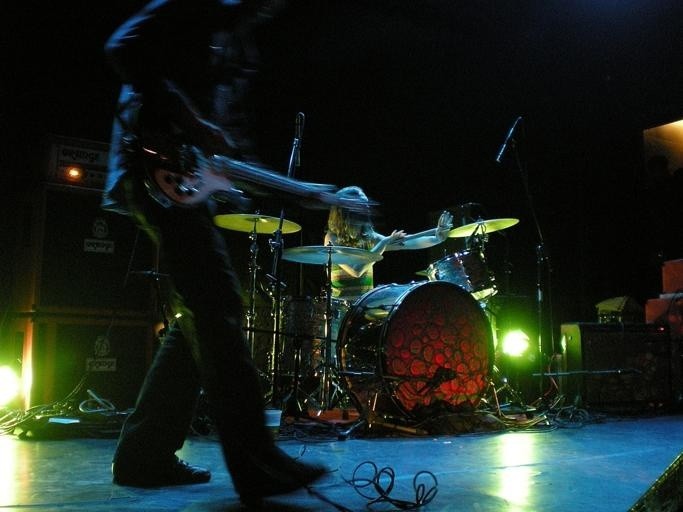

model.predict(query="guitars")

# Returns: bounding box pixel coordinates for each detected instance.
[144,149,380,213]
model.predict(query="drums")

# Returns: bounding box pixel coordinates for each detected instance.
[337,281,495,413]
[426,248,498,302]
[293,298,351,371]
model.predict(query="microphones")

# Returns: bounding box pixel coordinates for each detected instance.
[495,117,521,162]
[294,113,301,166]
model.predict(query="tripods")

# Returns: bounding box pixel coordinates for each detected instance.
[242,229,571,441]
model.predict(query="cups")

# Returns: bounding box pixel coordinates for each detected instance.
[262,408,283,438]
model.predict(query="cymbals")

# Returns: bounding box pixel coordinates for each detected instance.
[446,217,518,238]
[281,245,384,267]
[214,213,301,237]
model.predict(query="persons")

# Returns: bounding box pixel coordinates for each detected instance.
[99,1,328,503]
[322,184,455,363]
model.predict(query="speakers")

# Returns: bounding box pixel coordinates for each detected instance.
[45,317,154,420]
[34,184,155,318]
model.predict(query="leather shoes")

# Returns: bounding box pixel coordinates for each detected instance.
[112,454,211,489]
[236,455,332,504]
